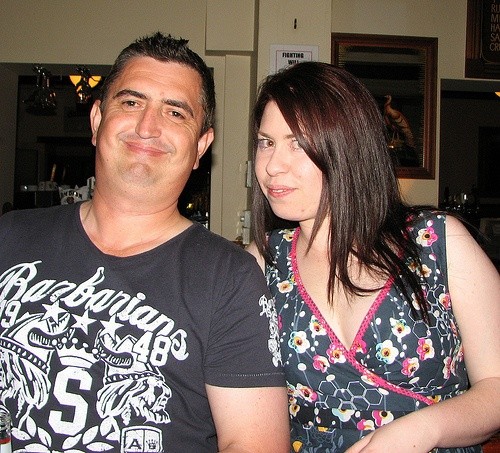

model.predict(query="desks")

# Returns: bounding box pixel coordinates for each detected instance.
[16,190,57,207]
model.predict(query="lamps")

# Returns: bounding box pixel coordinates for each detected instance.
[23,65,58,110]
[69,66,103,114]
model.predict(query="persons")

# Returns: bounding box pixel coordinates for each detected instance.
[247,62,500,453]
[0,31,292,453]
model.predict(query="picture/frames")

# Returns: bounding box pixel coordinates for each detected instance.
[465,0,500,79]
[331,32,436,178]
